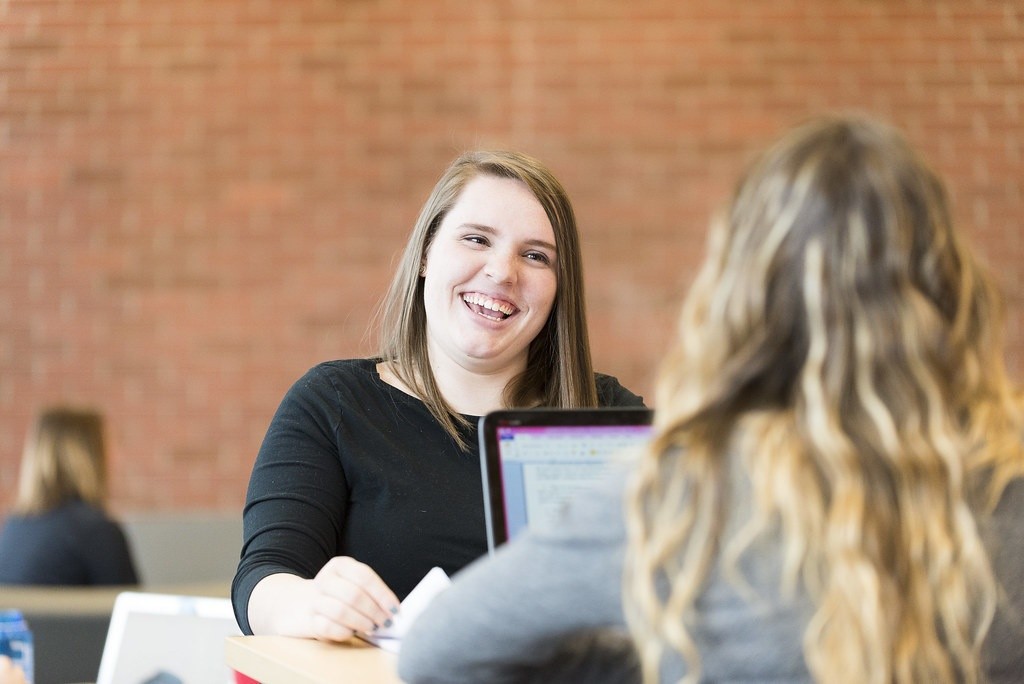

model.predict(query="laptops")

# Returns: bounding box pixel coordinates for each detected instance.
[477,407,655,553]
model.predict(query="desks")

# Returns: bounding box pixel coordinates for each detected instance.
[231,634,404,684]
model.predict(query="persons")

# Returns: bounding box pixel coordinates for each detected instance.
[0,404,144,587]
[394,109,1024,684]
[231,151,653,641]
